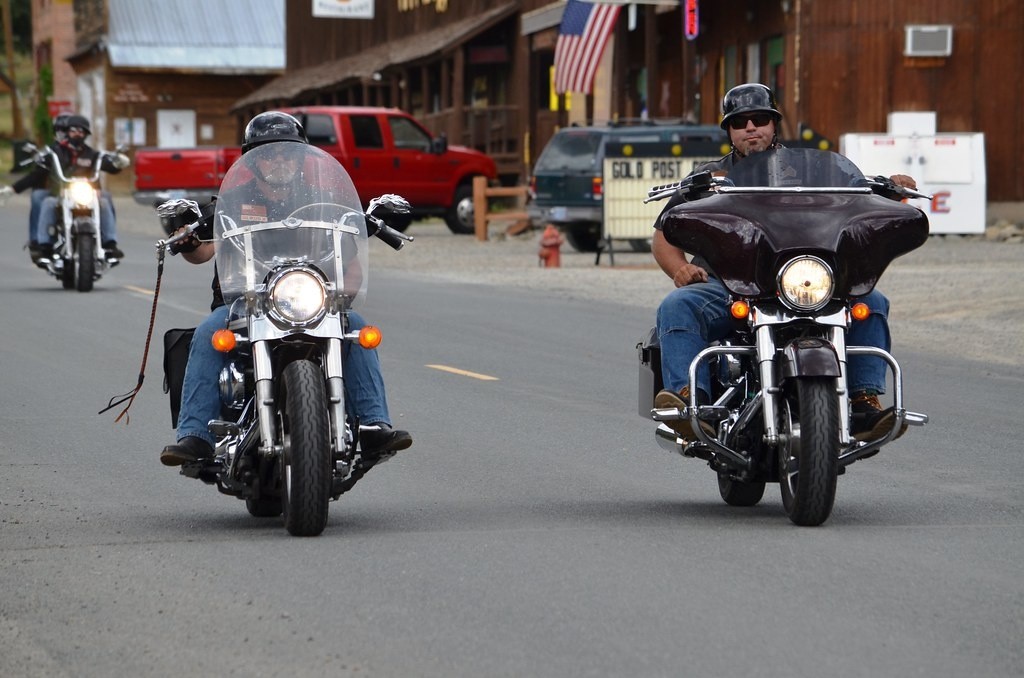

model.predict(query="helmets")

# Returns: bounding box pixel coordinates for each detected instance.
[52,116,70,128]
[241,111,308,155]
[721,83,783,131]
[66,116,90,134]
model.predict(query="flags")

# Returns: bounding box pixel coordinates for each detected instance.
[554,0,622,94]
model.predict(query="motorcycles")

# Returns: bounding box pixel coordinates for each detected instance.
[640,165,931,529]
[151,140,414,537]
[18,140,134,294]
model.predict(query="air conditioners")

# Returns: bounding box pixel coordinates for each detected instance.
[902,24,953,56]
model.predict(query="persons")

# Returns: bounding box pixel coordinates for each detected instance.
[160,111,413,466]
[652,83,916,444]
[0,113,130,263]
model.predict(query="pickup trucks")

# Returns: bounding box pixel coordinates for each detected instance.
[129,103,502,237]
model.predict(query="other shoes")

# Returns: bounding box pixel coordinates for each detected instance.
[30,240,53,262]
[103,241,123,258]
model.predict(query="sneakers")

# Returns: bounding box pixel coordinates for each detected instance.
[850,390,908,442]
[160,436,213,466]
[655,386,716,442]
[359,422,412,455]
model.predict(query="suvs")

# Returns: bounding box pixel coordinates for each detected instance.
[525,115,751,253]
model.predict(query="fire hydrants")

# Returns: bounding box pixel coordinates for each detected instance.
[538,222,565,271]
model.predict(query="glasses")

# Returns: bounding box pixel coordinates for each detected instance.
[54,126,66,132]
[69,126,85,133]
[729,112,772,129]
